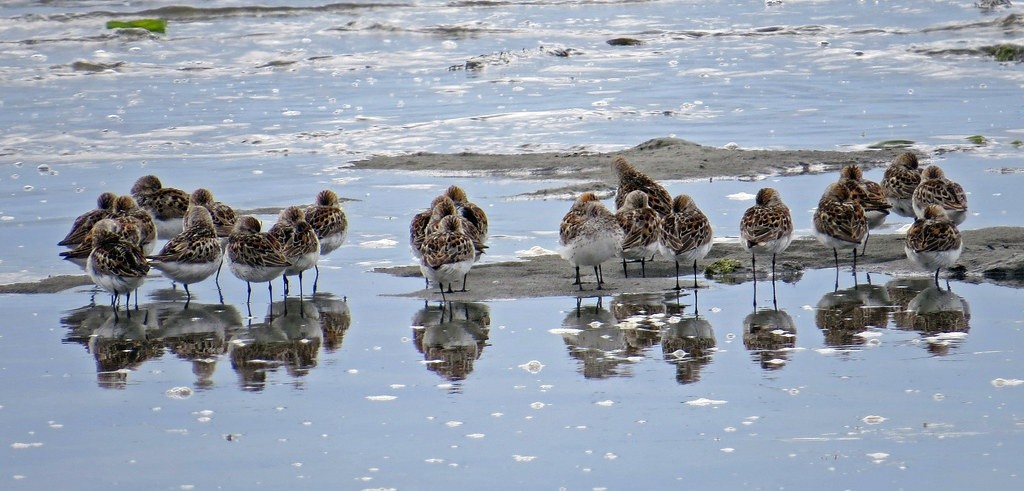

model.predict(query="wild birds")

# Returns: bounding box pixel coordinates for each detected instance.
[61,287,969,389]
[59,154,971,323]
